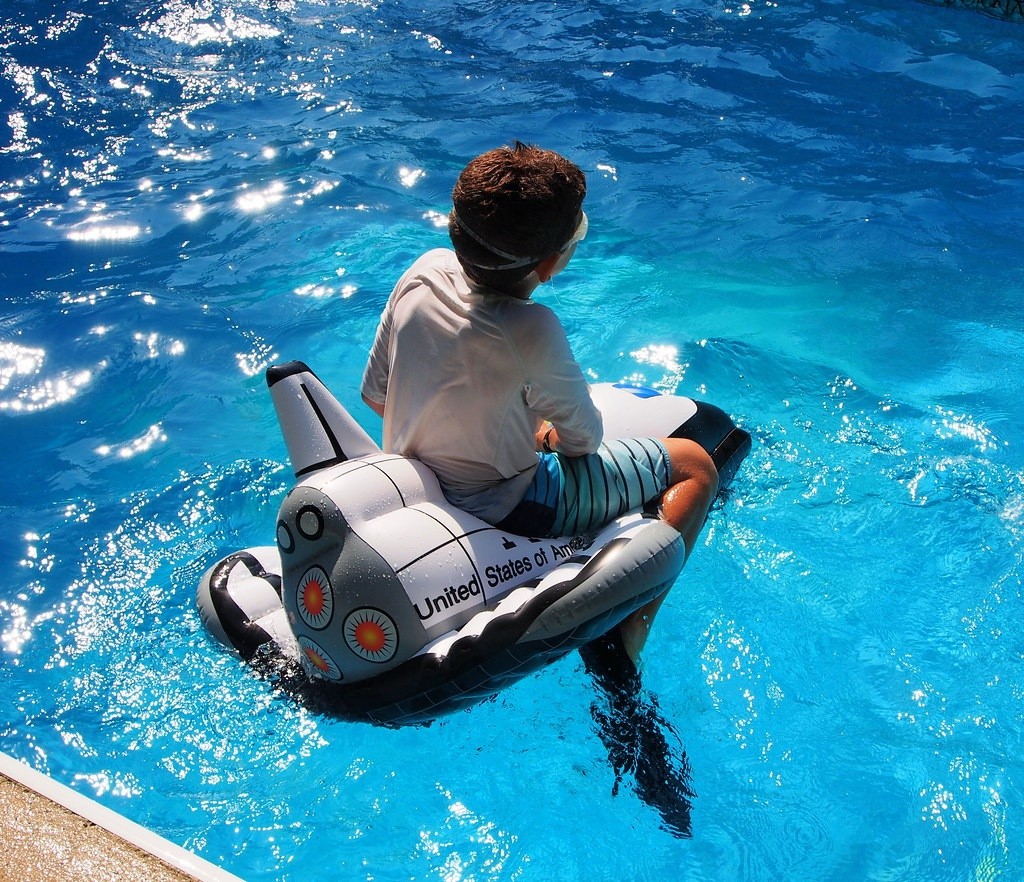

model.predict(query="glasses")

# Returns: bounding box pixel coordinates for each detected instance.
[559,211,588,252]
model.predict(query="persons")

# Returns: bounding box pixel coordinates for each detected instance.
[358,141,721,702]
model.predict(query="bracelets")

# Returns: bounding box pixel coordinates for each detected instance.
[541,424,559,454]
